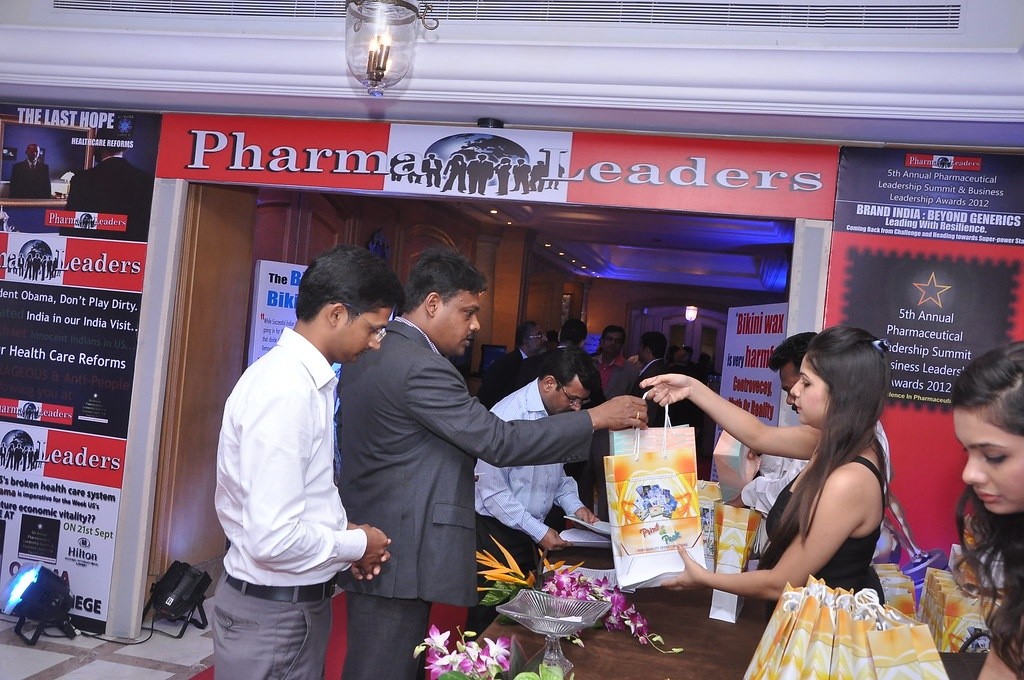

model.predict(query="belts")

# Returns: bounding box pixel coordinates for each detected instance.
[226,574,336,603]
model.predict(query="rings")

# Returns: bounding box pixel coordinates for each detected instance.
[637,412,639,419]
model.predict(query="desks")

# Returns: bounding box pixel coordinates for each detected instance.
[474,549,773,680]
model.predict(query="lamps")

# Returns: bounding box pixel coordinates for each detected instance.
[0,561,77,647]
[343,0,439,97]
[142,560,213,639]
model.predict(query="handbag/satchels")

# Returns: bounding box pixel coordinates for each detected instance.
[708,501,762,624]
[603,391,708,594]
[743,562,1009,680]
[697,479,721,573]
[608,392,696,470]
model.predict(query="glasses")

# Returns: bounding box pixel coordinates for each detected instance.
[330,302,386,342]
[555,375,591,406]
[528,334,542,339]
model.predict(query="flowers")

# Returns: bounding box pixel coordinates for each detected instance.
[412,624,541,680]
[475,533,685,655]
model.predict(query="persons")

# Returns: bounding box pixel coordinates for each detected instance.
[337,246,648,680]
[639,325,893,624]
[58,123,136,237]
[464,346,600,643]
[8,144,51,199]
[951,340,1024,680]
[212,242,406,680]
[476,319,711,533]
